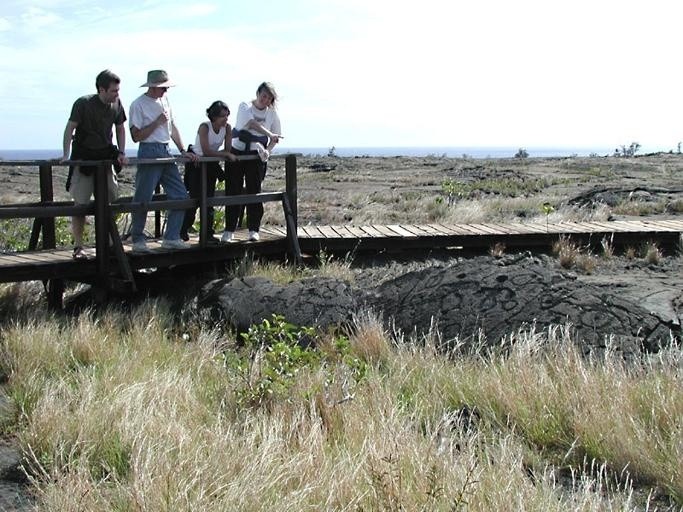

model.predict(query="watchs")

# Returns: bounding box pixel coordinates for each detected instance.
[180,148,186,152]
[120,151,125,155]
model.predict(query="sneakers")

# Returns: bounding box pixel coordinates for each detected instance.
[161,238,192,250]
[220,229,242,244]
[71,246,88,260]
[198,233,219,244]
[131,238,151,254]
[247,230,261,242]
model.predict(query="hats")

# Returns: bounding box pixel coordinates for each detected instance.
[139,70,177,88]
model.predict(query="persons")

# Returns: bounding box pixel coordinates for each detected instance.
[58,70,127,262]
[129,69,200,252]
[220,81,284,244]
[180,100,240,244]
